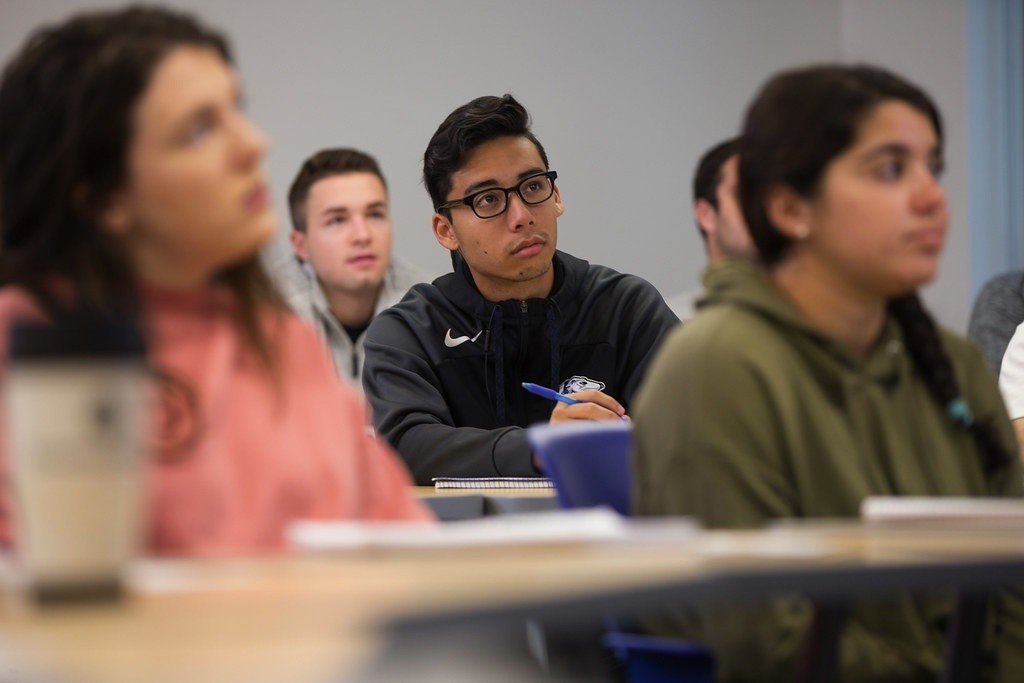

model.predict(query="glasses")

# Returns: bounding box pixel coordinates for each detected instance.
[436,171,557,218]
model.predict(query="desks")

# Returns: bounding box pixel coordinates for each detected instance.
[0,488,1024,683]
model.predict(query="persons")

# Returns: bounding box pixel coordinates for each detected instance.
[636,64,1024,683]
[0,7,439,565]
[277,90,1024,487]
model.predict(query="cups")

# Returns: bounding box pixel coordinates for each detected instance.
[7,358,147,606]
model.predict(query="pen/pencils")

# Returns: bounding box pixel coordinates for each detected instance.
[521,381,578,405]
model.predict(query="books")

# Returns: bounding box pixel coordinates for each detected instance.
[430,476,554,489]
